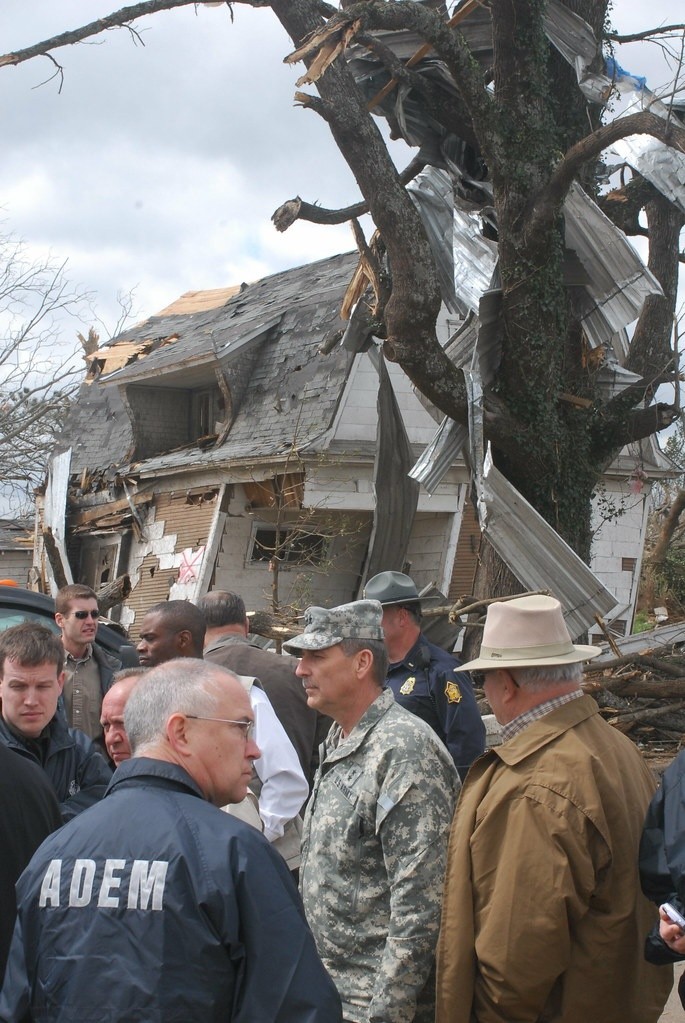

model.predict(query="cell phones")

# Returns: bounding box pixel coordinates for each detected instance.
[662,903,685,938]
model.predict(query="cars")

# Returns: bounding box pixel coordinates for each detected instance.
[0,580,140,656]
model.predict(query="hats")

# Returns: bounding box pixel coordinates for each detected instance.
[283,598,384,656]
[453,595,602,672]
[363,571,440,606]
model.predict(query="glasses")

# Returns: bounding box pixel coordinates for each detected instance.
[474,669,520,689]
[168,715,254,742]
[61,610,100,619]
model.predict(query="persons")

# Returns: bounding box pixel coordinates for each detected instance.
[434,596,675,1023]
[0,622,113,825]
[282,598,463,1023]
[0,657,343,1023]
[100,667,153,768]
[195,591,316,782]
[135,600,310,876]
[54,583,127,752]
[362,570,488,772]
[636,747,685,1008]
